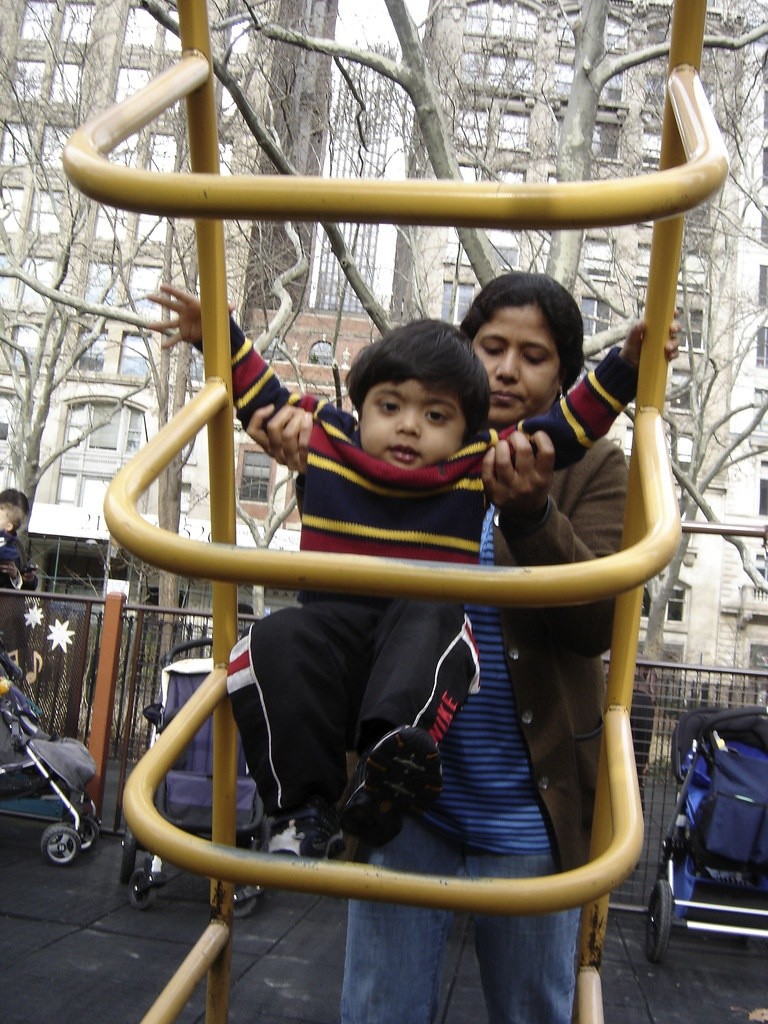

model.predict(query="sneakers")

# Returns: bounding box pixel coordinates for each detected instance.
[260,804,347,862]
[339,725,444,851]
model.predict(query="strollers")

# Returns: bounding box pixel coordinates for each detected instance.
[126,638,276,919]
[643,706,768,966]
[0,626,103,866]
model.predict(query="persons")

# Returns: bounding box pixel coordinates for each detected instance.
[146,283,679,864]
[0,488,38,693]
[247,273,629,1024]
